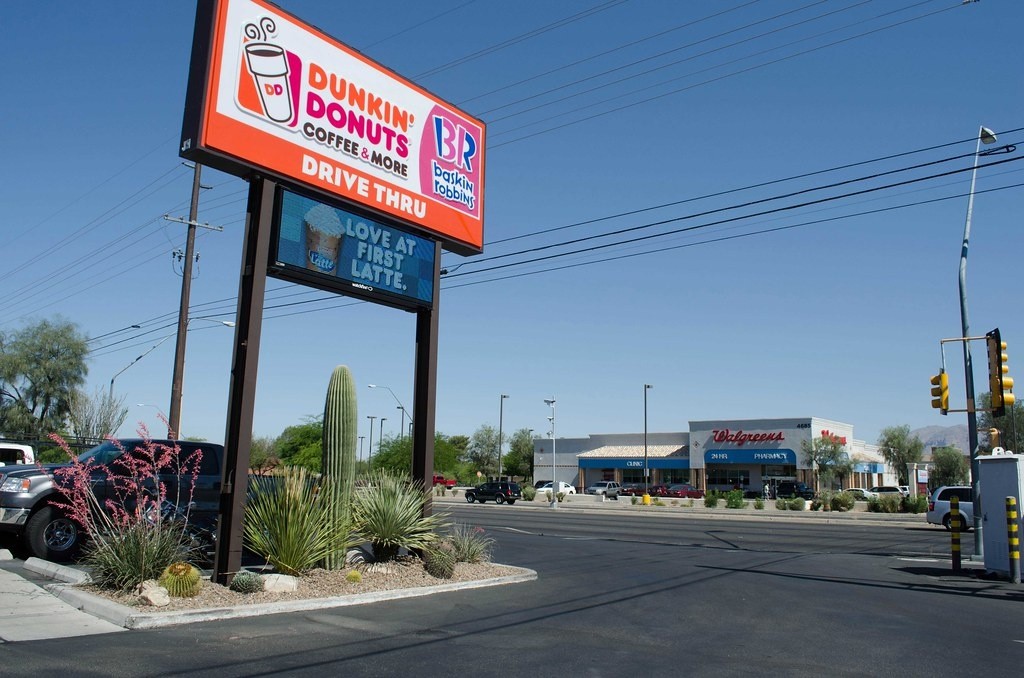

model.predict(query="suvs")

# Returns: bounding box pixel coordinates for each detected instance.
[465,480,521,505]
[776,481,814,500]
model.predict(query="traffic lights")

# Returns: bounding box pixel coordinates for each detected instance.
[985,328,1015,418]
[930,374,949,410]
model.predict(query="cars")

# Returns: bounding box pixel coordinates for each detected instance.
[843,485,909,501]
[622,484,705,498]
[926,486,974,531]
[584,481,622,500]
[535,480,576,497]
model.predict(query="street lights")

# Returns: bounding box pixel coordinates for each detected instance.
[644,383,653,497]
[498,394,509,482]
[397,407,403,441]
[957,125,998,556]
[379,418,387,451]
[358,436,365,467]
[367,384,413,421]
[109,316,236,411]
[367,415,377,466]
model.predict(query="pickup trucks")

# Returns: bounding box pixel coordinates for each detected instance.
[433,475,457,489]
[0,439,320,566]
